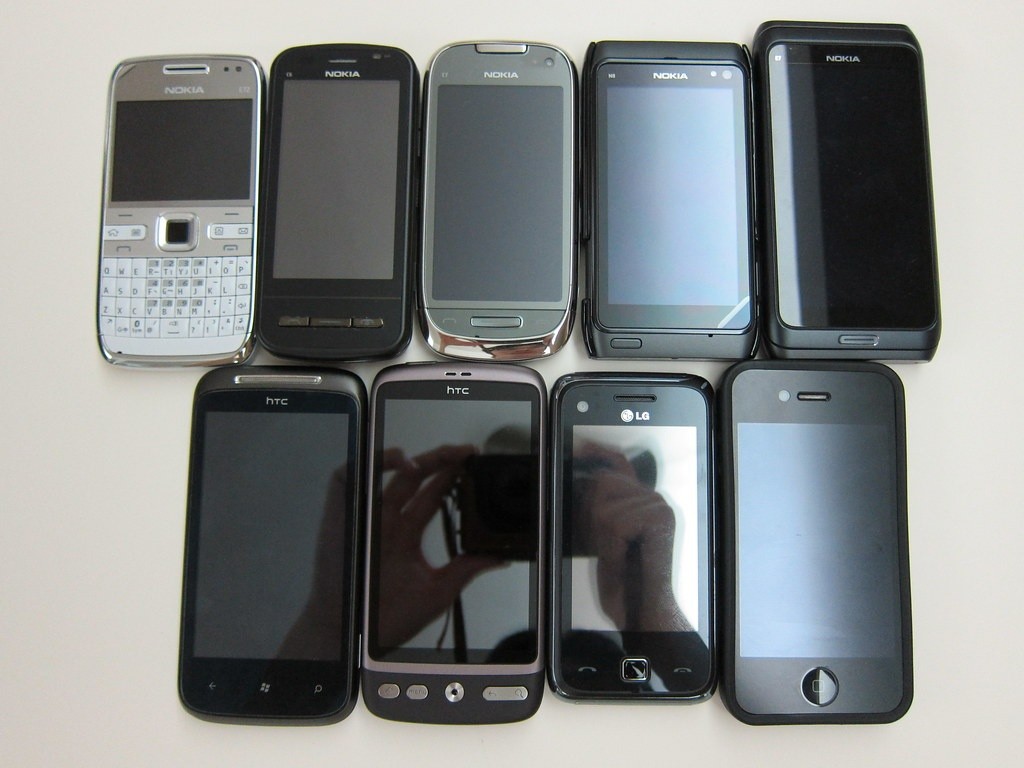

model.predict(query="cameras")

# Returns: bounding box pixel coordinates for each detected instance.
[454,452,602,563]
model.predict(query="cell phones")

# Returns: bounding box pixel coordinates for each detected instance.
[95,20,941,726]
